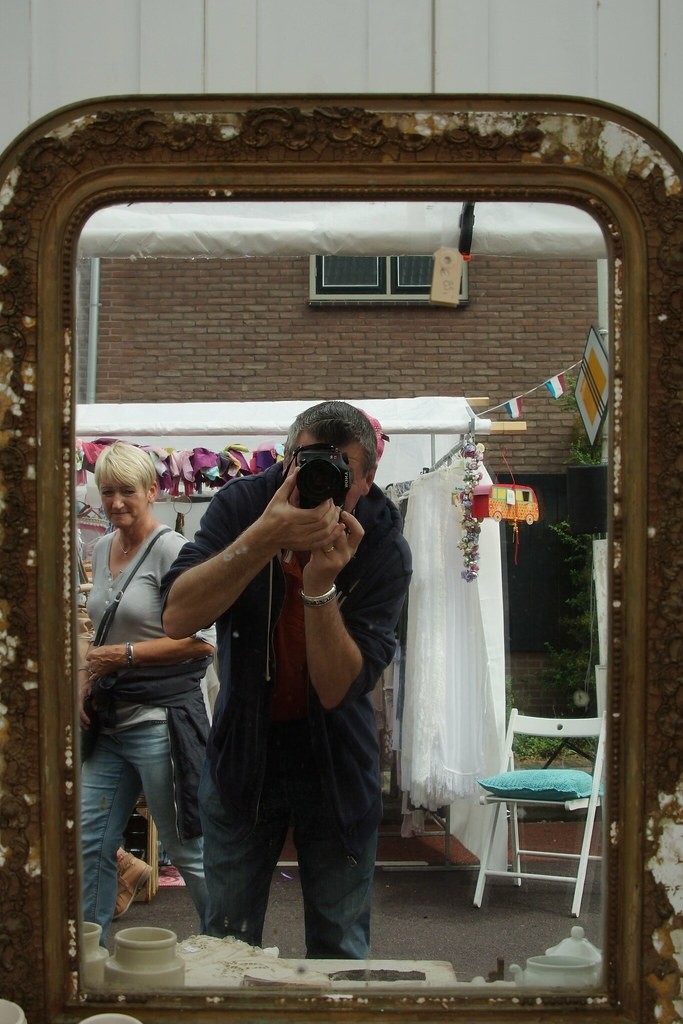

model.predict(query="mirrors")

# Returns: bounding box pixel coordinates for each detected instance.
[0,92,683,1024]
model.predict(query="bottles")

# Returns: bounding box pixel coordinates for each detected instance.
[510,956,599,987]
[78,1012,142,1024]
[0,999,28,1024]
[82,922,109,989]
[103,926,185,990]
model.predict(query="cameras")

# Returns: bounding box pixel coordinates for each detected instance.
[296,444,353,523]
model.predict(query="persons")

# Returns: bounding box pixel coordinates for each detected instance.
[74,441,218,956]
[159,401,414,960]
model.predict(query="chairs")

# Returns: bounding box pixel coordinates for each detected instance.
[472,708,606,919]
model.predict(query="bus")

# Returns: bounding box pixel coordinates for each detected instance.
[471,483,540,526]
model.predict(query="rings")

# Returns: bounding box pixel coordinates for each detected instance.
[328,546,334,552]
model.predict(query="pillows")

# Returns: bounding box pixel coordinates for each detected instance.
[476,768,604,800]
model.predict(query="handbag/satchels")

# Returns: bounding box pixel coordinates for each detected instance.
[80,687,114,747]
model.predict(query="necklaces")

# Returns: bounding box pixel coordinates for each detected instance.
[118,539,137,555]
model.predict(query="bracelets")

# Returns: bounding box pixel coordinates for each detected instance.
[301,584,337,607]
[127,642,134,668]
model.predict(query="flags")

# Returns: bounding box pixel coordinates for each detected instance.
[545,374,566,400]
[504,396,522,419]
